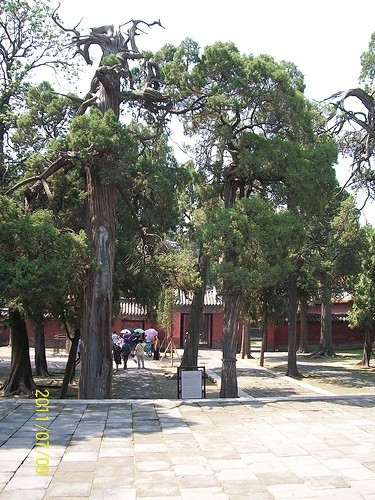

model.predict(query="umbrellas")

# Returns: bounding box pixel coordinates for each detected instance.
[134,329,144,334]
[145,328,159,337]
[120,329,131,334]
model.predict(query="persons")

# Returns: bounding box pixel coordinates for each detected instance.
[77,332,162,371]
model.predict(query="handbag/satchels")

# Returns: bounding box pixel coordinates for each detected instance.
[133,356,137,363]
